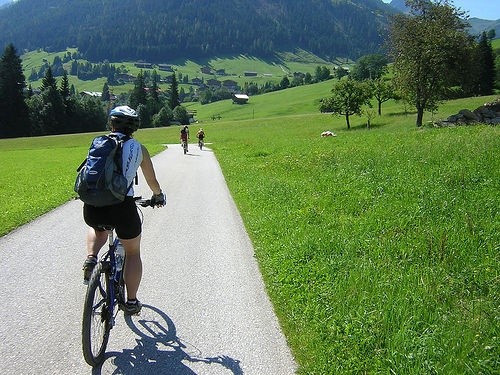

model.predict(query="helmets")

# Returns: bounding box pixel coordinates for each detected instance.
[109,105,140,130]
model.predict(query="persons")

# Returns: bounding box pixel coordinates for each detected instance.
[196,128,206,147]
[81,106,166,316]
[180,125,190,152]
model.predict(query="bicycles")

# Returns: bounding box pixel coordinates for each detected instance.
[181,138,190,154]
[72,194,167,366]
[198,137,204,151]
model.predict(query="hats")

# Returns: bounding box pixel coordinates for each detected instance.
[185,126,188,128]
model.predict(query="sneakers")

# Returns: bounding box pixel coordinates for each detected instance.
[125,300,142,315]
[83,255,97,281]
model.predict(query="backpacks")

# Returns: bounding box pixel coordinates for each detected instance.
[74,133,133,206]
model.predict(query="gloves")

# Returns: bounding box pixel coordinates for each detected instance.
[150,190,167,209]
